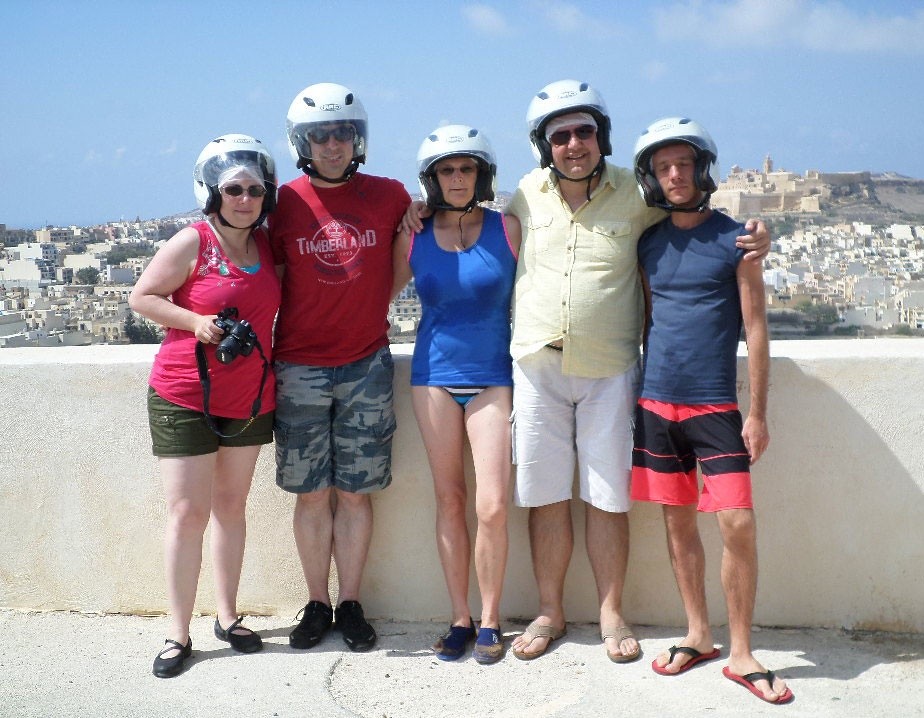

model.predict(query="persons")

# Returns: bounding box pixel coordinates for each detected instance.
[396,80,771,662]
[128,134,284,680]
[267,84,411,652]
[632,118,793,705]
[389,124,521,662]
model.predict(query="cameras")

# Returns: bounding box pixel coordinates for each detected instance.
[214,315,258,365]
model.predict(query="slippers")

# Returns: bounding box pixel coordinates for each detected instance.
[722,663,793,704]
[598,625,640,663]
[511,620,568,661]
[651,642,722,676]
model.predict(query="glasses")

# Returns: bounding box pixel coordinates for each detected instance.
[547,125,596,145]
[222,184,267,197]
[436,164,480,176]
[305,126,354,144]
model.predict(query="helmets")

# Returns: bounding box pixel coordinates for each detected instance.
[285,83,368,163]
[525,79,613,169]
[632,118,718,207]
[416,126,495,208]
[192,134,279,216]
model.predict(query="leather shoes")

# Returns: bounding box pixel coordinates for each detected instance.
[213,613,264,654]
[153,638,193,679]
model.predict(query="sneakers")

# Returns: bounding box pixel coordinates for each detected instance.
[473,624,505,665]
[289,601,334,649]
[334,600,377,652]
[436,615,477,660]
